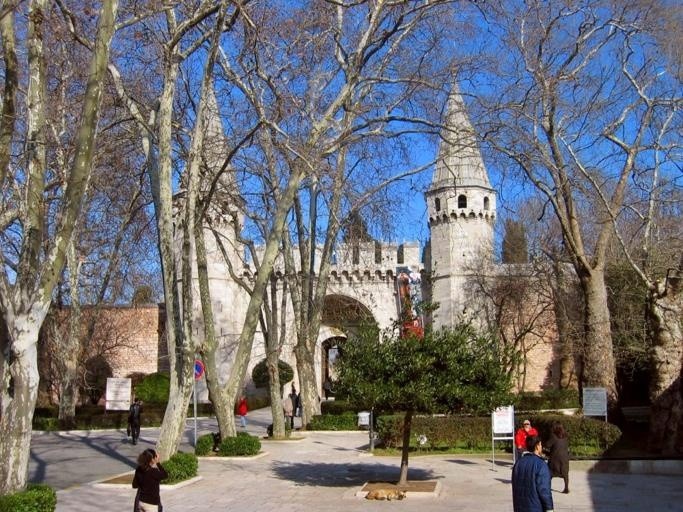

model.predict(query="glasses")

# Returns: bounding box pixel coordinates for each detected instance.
[523,424,529,425]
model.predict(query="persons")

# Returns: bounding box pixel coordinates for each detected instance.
[236,390,248,429]
[126,397,143,446]
[291,381,296,394]
[296,392,302,417]
[513,419,538,461]
[509,435,555,512]
[543,420,571,496]
[283,393,296,429]
[128,448,167,512]
[280,395,285,408]
[289,389,297,418]
[321,378,330,401]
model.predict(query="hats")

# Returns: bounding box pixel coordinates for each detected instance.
[133,398,140,402]
[523,420,530,424]
